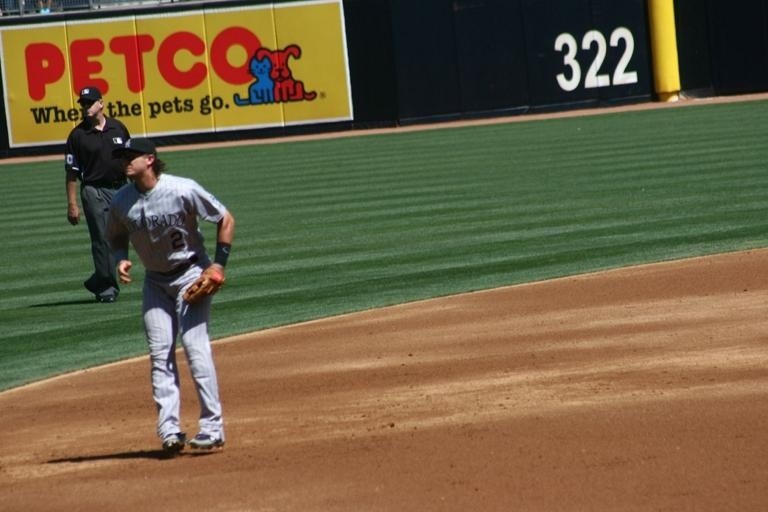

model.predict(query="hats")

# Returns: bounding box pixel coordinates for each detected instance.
[78,86,100,104]
[111,137,157,160]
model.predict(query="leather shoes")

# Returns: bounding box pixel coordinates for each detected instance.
[97,295,116,303]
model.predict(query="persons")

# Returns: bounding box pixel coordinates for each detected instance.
[63,86,131,304]
[105,136,237,458]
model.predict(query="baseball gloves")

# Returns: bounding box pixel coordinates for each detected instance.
[183,264,225,304]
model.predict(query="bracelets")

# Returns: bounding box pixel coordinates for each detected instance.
[213,240,232,267]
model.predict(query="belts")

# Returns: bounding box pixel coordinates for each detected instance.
[176,255,197,270]
[94,179,128,189]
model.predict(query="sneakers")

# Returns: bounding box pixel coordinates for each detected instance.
[163,438,185,452]
[190,435,224,450]
